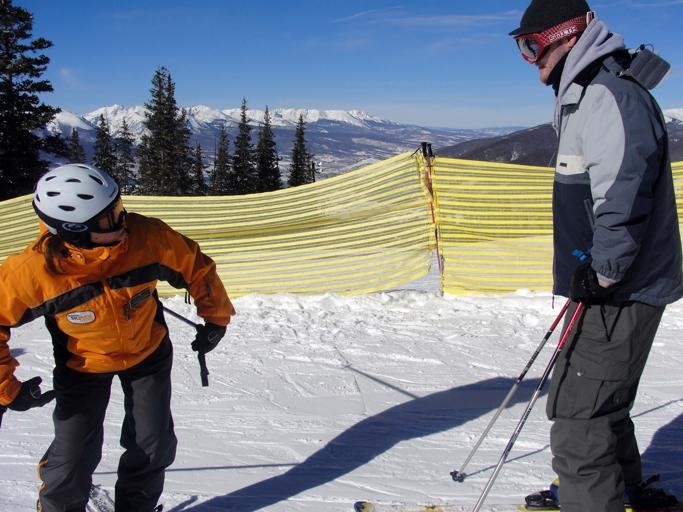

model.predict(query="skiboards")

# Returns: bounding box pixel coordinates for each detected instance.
[354,473,683,512]
[85,482,116,512]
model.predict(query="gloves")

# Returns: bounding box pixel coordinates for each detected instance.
[6,376,56,411]
[568,256,609,307]
[191,323,226,356]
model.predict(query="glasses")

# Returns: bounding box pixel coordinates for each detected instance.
[33,188,127,234]
[513,11,596,64]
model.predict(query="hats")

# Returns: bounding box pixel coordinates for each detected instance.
[509,0,590,36]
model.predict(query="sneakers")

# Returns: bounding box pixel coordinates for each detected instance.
[526,478,561,507]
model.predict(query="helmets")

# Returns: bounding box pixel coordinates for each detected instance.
[31,162,119,249]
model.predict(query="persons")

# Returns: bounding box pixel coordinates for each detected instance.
[509,1,682,510]
[0,164,237,508]
